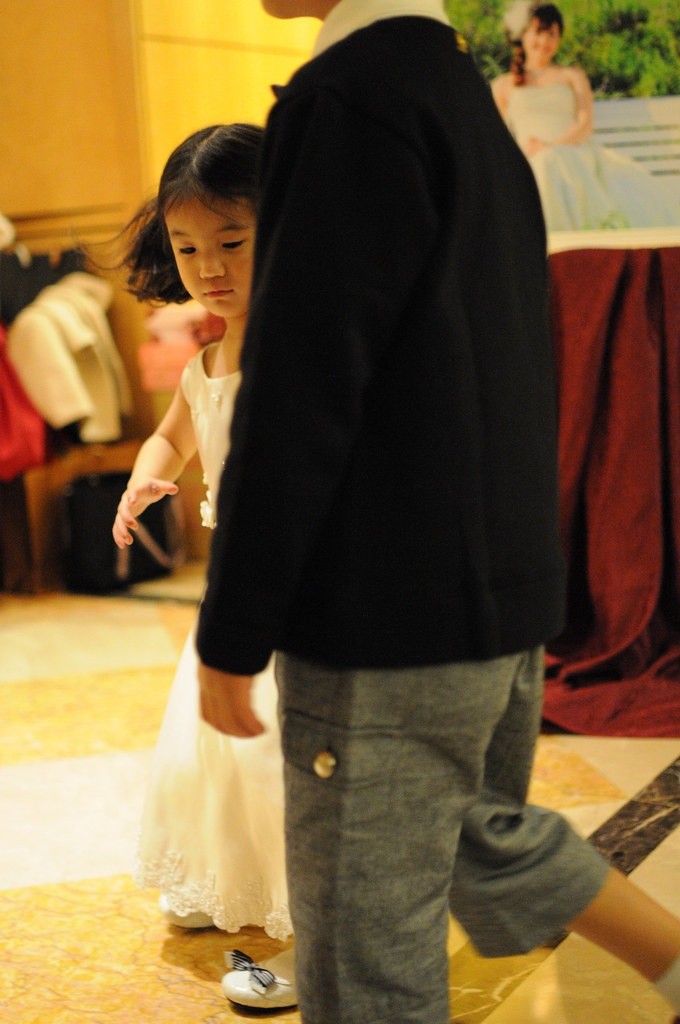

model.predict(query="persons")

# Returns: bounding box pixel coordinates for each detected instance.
[195,0,680,1024]
[111,123,299,1007]
[490,0,680,230]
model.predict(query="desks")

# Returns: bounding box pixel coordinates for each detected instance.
[546,232,680,742]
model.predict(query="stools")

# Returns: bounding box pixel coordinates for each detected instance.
[12,436,186,593]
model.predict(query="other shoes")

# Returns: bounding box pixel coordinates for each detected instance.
[221,949,297,1008]
[160,895,215,928]
